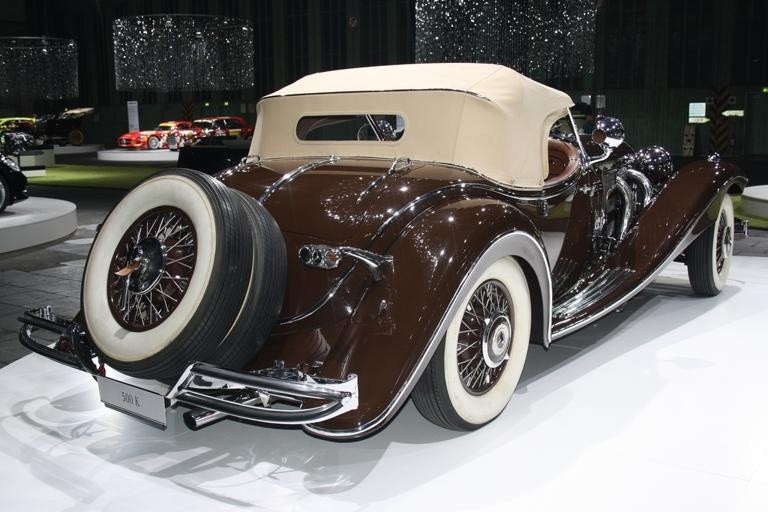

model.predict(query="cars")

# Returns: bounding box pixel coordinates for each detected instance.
[8,60,754,449]
[0,151,30,213]
[0,106,252,154]
